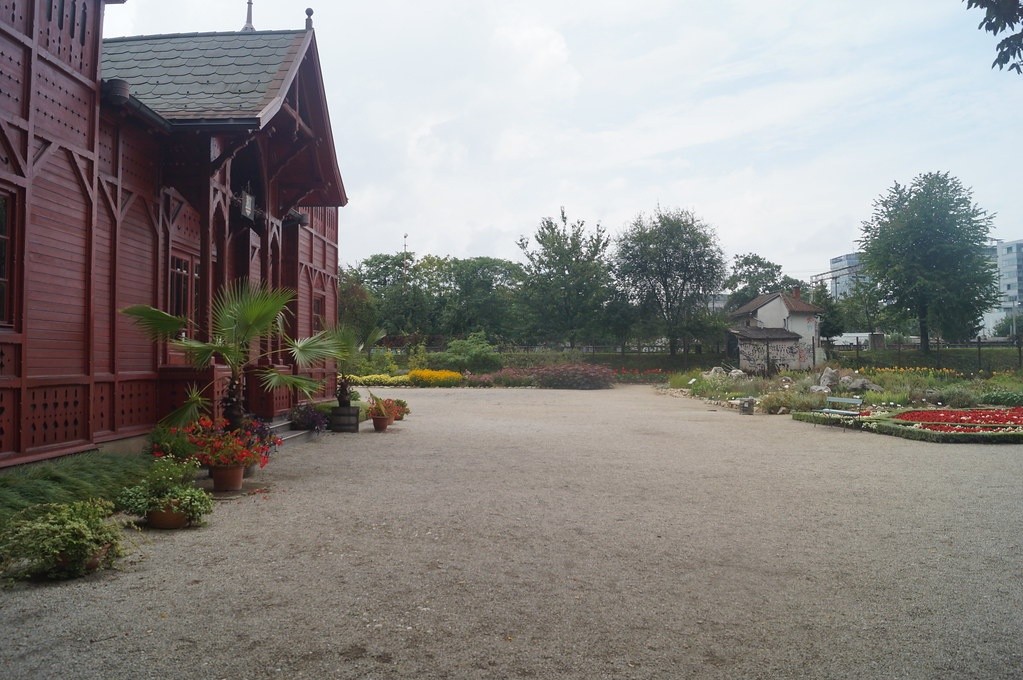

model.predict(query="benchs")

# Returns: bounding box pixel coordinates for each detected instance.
[811,397,864,433]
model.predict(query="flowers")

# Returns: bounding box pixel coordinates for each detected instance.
[365,399,411,418]
[115,453,214,527]
[153,417,284,469]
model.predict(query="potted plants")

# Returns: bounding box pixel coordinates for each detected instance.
[316,316,393,425]
[0,497,155,591]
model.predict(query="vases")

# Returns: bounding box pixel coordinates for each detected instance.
[387,416,394,424]
[394,413,403,420]
[147,499,190,529]
[371,416,389,431]
[210,464,246,491]
